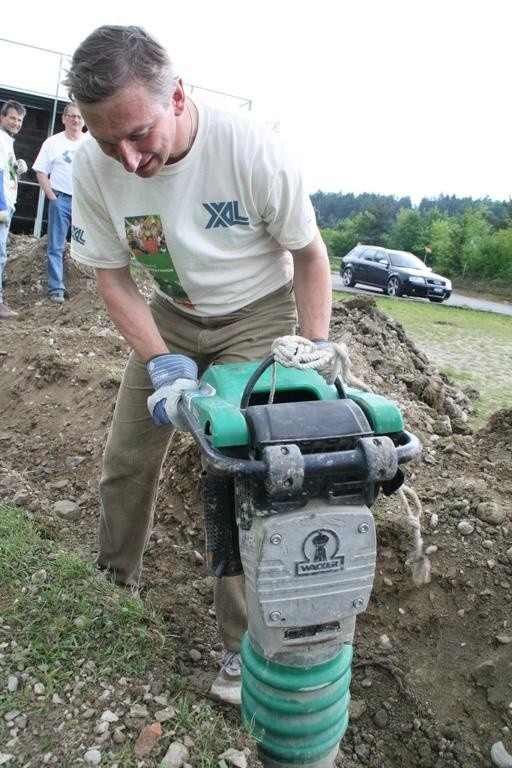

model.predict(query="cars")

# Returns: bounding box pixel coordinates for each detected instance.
[340,245,453,303]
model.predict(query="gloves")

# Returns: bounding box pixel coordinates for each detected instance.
[143,352,199,433]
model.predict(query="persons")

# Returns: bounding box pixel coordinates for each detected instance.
[32,102,95,308]
[59,22,335,708]
[0,97,29,320]
[122,214,170,257]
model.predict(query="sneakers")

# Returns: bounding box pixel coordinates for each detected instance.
[48,289,65,307]
[0,303,21,322]
[207,648,245,709]
